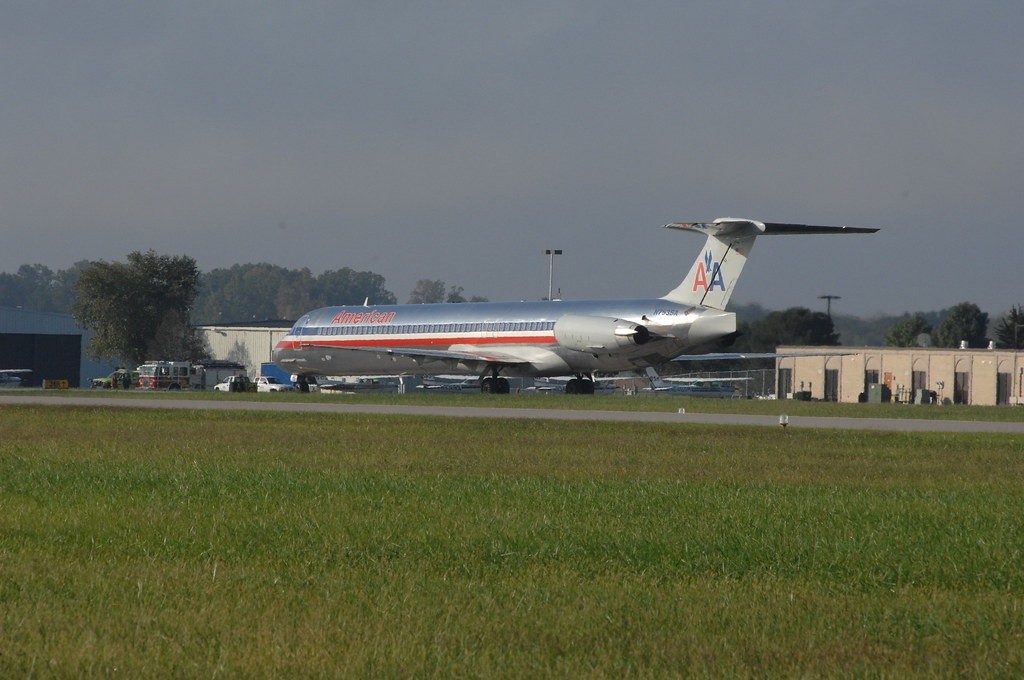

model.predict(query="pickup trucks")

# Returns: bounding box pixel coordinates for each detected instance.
[214,375,257,394]
[253,375,295,393]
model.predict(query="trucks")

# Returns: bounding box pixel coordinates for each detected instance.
[136,360,207,392]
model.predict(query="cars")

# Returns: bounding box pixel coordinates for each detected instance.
[90,368,141,390]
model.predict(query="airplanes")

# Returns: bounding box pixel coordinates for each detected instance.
[270,215,883,396]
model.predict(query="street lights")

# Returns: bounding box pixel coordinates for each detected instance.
[546,250,562,300]
